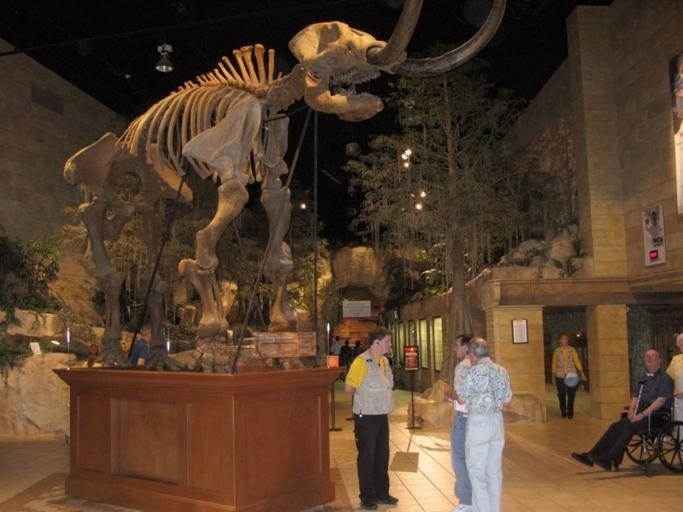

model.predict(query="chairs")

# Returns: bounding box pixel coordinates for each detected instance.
[378,495,398,504]
[360,500,378,510]
[587,453,612,471]
[572,451,594,467]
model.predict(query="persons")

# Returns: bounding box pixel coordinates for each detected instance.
[128,323,151,368]
[343,326,398,509]
[338,339,351,381]
[444,333,472,512]
[570,348,673,474]
[643,210,662,237]
[329,335,342,355]
[461,336,514,511]
[353,339,363,359]
[665,333,683,470]
[550,332,589,419]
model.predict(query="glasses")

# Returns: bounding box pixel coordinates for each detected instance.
[151,34,174,73]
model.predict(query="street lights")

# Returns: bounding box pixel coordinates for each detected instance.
[563,373,580,387]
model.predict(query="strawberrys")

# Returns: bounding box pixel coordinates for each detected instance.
[620,392,683,474]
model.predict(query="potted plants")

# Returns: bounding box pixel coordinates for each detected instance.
[666,47,683,214]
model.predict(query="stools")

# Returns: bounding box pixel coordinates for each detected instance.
[462,413,467,417]
